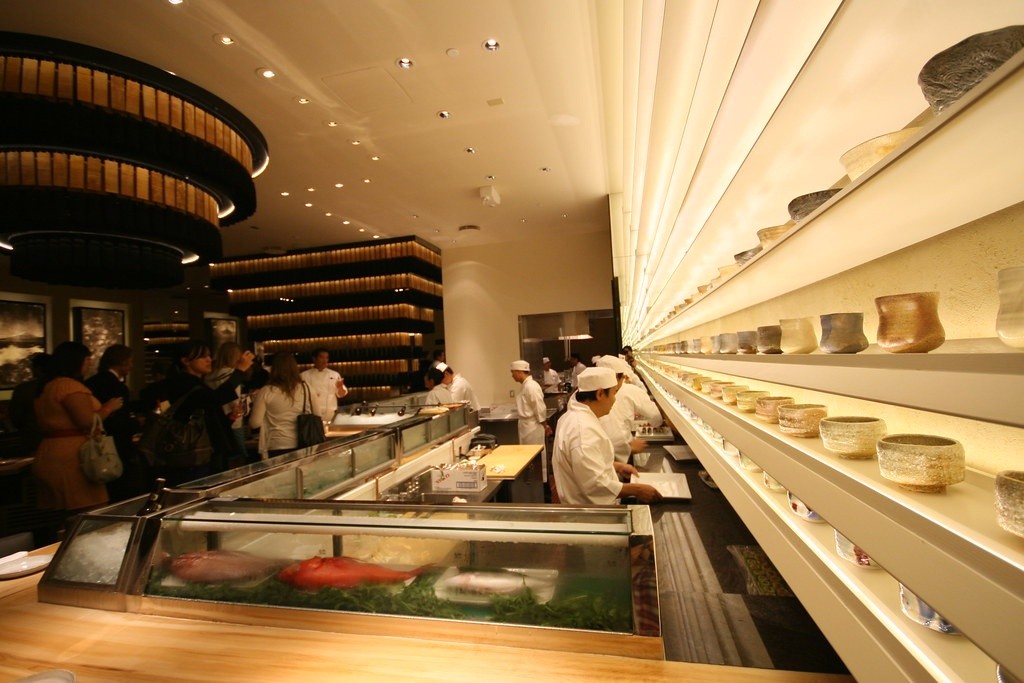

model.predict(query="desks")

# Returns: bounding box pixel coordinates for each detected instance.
[475,439,545,504]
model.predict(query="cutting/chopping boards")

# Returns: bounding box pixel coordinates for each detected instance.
[475,444,544,477]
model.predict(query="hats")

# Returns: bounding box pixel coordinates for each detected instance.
[577,365,618,393]
[598,355,627,373]
[592,356,601,364]
[511,360,530,372]
[543,357,550,364]
[436,362,448,373]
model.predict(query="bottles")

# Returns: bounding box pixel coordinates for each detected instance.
[351,401,407,417]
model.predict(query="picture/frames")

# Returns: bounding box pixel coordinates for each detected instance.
[205,319,238,361]
[72,306,126,379]
[0,300,47,387]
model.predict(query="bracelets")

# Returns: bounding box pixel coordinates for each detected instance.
[102,406,112,413]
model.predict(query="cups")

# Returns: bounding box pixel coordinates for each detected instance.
[819,313,869,354]
[687,326,782,354]
[995,265,1024,348]
[779,317,818,355]
[875,292,945,353]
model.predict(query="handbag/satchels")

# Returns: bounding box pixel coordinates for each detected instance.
[156,388,212,466]
[79,414,124,485]
[297,380,326,448]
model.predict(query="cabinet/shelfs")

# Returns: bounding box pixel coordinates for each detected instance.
[620,0,1023,683]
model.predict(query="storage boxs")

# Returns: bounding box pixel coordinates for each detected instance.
[432,460,489,491]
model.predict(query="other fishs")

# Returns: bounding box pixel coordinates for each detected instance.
[275,554,444,595]
[443,569,556,597]
[169,548,305,582]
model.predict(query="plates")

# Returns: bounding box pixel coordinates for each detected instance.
[631,416,675,441]
[629,472,692,499]
[16,669,77,683]
[0,556,53,578]
[663,445,697,460]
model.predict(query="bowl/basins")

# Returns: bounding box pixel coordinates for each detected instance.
[876,432,966,493]
[778,403,827,438]
[635,453,650,467]
[673,370,793,424]
[734,247,769,268]
[995,470,1024,539]
[788,188,849,223]
[840,126,931,182]
[756,225,800,250]
[674,264,740,313]
[818,414,888,458]
[917,25,1024,118]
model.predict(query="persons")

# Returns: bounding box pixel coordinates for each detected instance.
[510,360,552,504]
[436,362,481,411]
[570,350,587,386]
[542,357,563,392]
[428,350,445,368]
[8,342,349,524]
[424,368,451,404]
[551,346,665,505]
